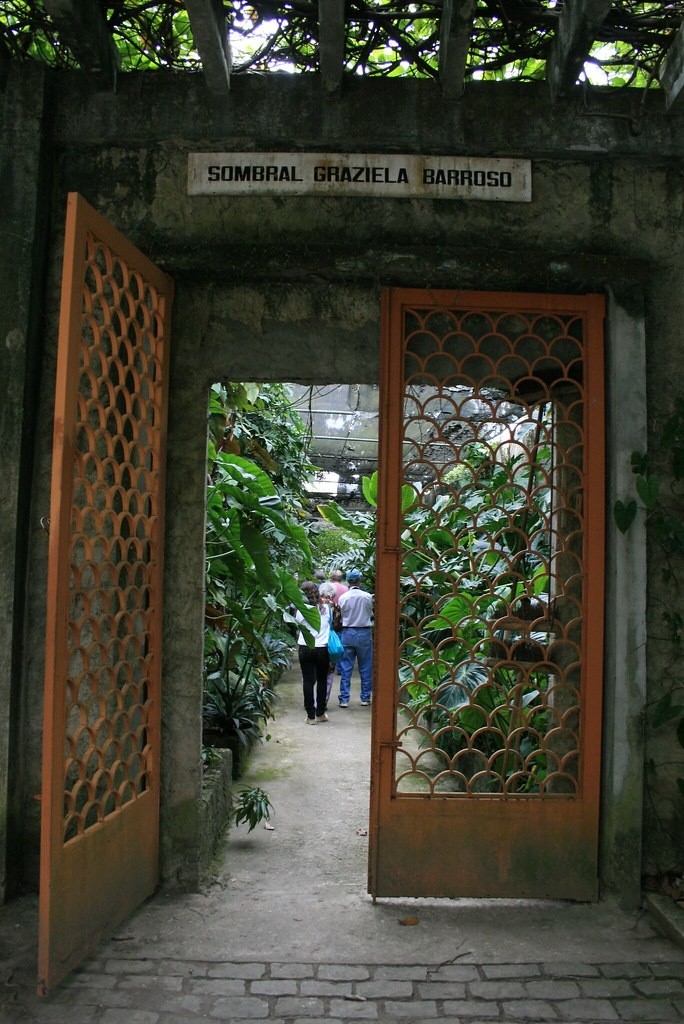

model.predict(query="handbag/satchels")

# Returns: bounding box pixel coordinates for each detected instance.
[326,604,344,662]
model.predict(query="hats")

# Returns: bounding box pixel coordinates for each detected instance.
[346,568,362,583]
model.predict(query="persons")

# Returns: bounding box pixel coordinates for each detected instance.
[290,568,348,723]
[338,569,374,708]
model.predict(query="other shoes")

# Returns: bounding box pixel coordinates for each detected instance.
[316,714,329,721]
[305,718,316,725]
[339,703,348,707]
[361,702,371,706]
